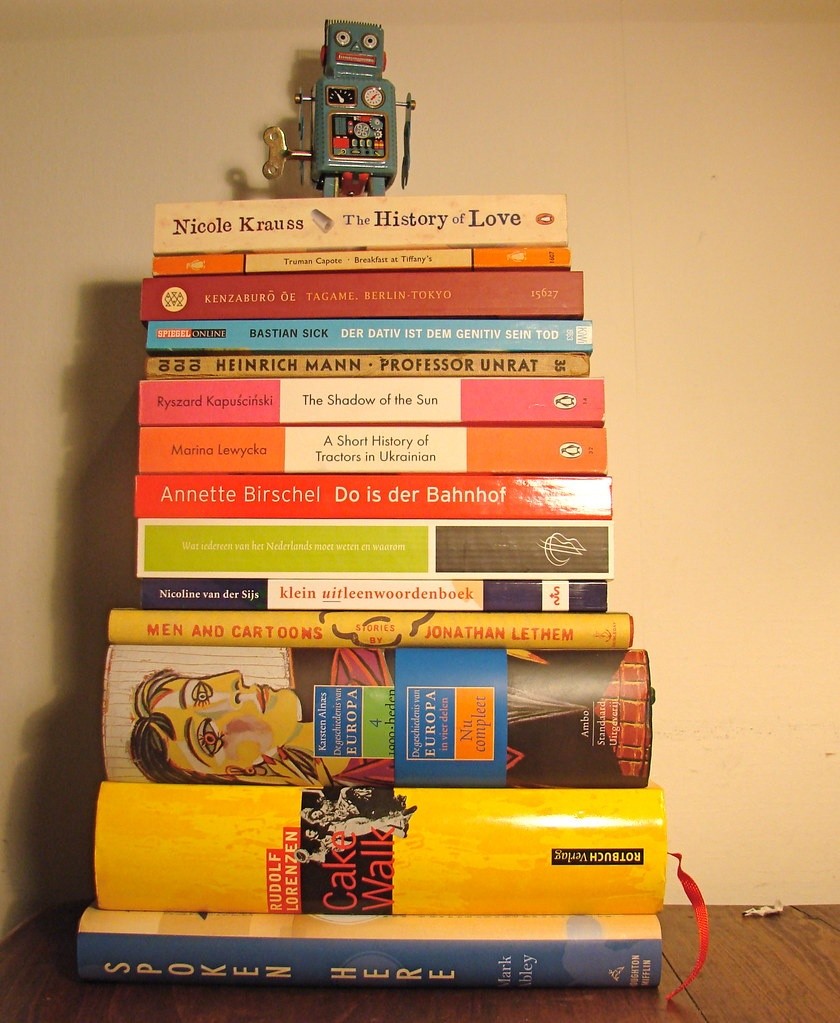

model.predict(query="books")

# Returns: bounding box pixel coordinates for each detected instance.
[76,193,667,987]
